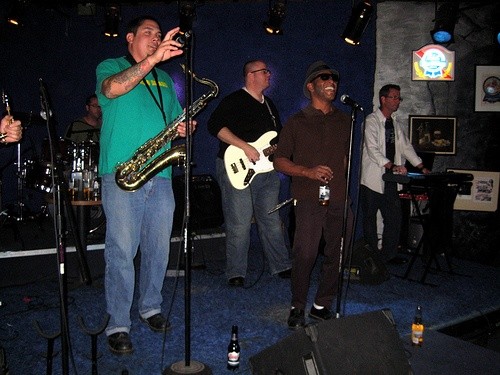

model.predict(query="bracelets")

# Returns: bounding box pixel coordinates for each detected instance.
[420,165,427,169]
[390,164,397,171]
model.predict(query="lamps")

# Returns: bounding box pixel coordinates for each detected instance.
[176,0,197,36]
[344,0,375,46]
[262,0,288,35]
[430,0,461,44]
[103,4,123,37]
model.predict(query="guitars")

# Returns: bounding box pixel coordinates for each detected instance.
[223,130,278,191]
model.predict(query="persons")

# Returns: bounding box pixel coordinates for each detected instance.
[62,97,101,153]
[273,60,353,330]
[207,60,292,286]
[0,114,23,143]
[95,16,197,355]
[358,84,430,265]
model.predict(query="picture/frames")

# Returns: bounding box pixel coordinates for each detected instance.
[473,65,500,112]
[406,114,456,155]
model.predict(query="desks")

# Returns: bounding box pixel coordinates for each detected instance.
[71,198,100,282]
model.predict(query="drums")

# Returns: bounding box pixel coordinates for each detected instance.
[66,171,102,206]
[24,155,60,194]
[60,139,96,173]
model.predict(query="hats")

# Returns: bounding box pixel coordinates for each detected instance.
[303,61,339,99]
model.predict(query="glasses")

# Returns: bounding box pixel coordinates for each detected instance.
[251,69,272,75]
[88,104,101,108]
[385,95,404,102]
[309,72,339,83]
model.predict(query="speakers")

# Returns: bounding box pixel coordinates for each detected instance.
[248,307,415,375]
[173,174,225,232]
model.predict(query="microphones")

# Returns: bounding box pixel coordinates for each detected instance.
[66,122,74,138]
[176,29,194,47]
[341,94,363,110]
[39,78,52,120]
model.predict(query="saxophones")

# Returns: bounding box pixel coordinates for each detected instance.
[114,62,219,191]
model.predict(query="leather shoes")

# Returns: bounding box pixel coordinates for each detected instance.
[146,314,171,331]
[382,246,412,255]
[278,268,292,279]
[108,333,132,353]
[309,304,335,320]
[386,256,409,265]
[287,307,305,329]
[229,277,244,287]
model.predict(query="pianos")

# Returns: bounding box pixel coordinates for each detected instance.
[382,171,475,289]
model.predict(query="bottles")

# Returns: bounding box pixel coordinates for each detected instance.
[412,306,425,348]
[227,325,240,371]
[318,177,330,207]
